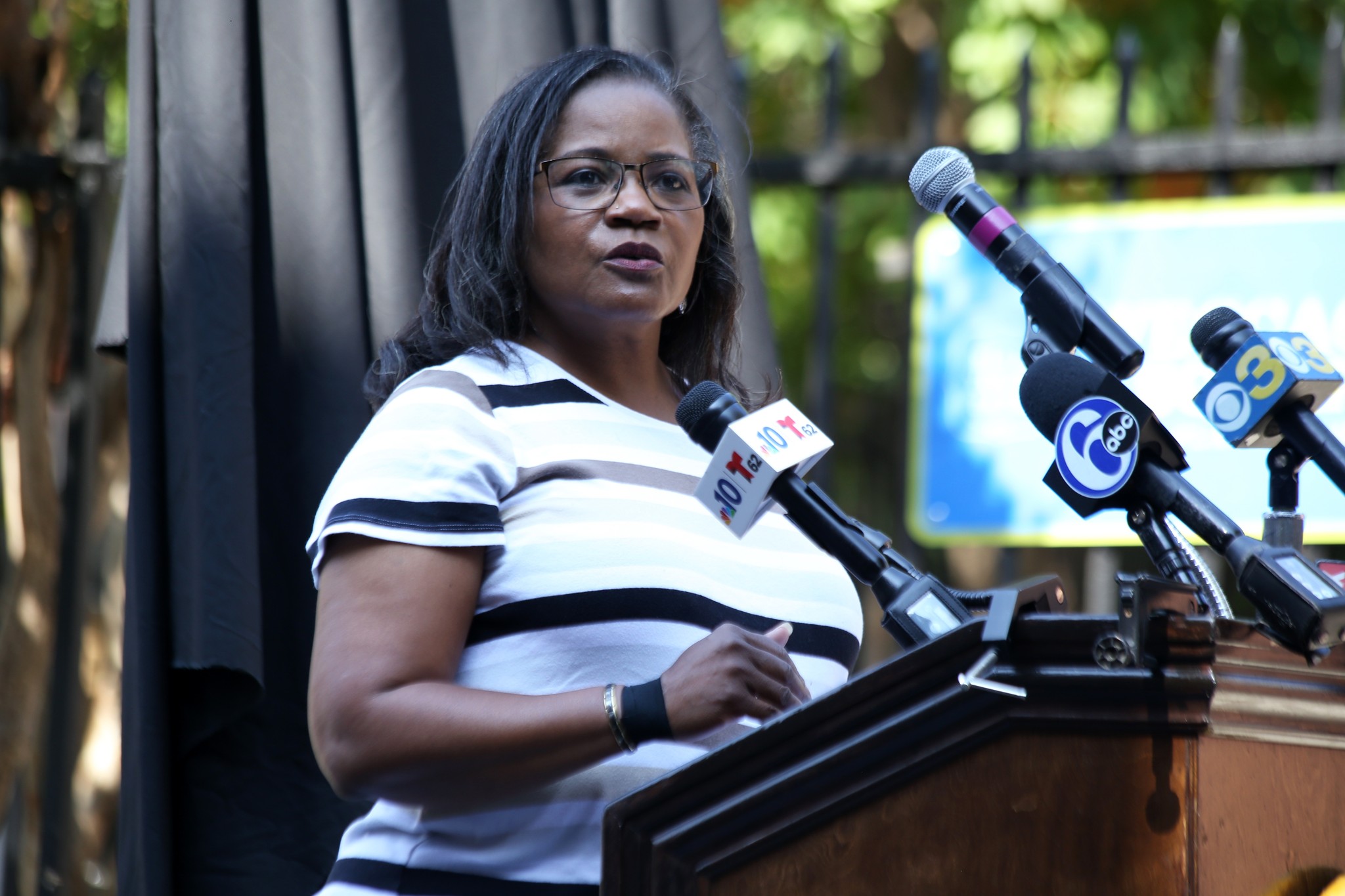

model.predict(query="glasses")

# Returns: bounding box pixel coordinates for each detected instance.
[534,157,718,211]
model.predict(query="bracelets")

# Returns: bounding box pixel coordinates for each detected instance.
[603,683,638,756]
[620,677,676,748]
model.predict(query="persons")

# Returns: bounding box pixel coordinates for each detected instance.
[304,45,866,896]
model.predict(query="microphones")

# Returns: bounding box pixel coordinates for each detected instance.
[908,144,1144,380]
[1019,352,1345,658]
[674,380,974,653]
[1191,306,1345,494]
